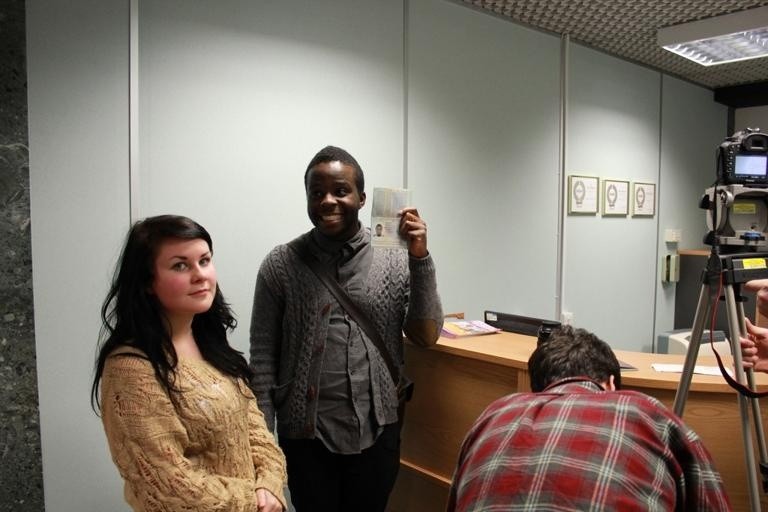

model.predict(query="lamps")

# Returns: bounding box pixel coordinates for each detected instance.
[656,5,767,66]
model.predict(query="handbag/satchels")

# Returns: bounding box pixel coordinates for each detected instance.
[393,364,414,427]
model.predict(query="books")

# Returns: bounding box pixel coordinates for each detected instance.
[441,321,501,338]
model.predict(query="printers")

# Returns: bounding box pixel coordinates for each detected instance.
[658,329,732,358]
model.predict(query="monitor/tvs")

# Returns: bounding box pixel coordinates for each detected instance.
[484,310,560,337]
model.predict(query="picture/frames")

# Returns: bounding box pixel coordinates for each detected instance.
[601,179,629,216]
[567,174,598,214]
[630,181,655,216]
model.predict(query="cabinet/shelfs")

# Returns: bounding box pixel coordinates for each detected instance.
[674,250,767,337]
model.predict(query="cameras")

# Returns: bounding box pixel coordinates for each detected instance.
[714,126,768,189]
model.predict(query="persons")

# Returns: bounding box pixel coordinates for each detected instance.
[739,278,768,373]
[250,146,444,511]
[101,215,288,511]
[446,325,733,512]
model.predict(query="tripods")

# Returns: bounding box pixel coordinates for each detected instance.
[669,241,768,512]
